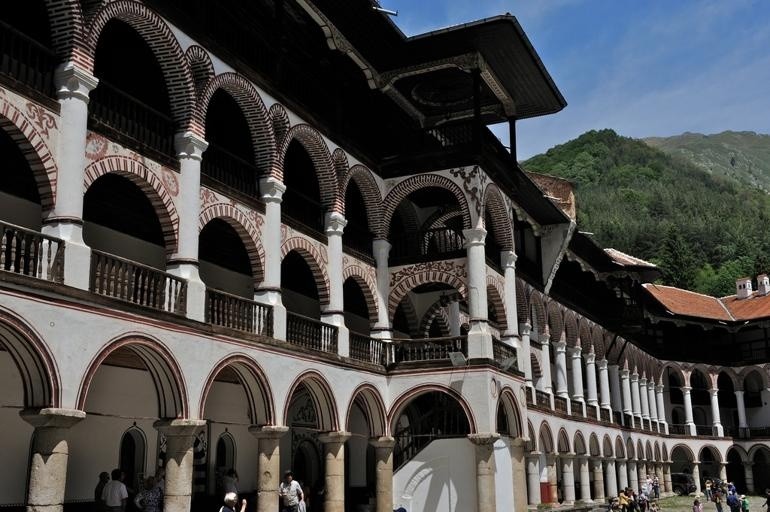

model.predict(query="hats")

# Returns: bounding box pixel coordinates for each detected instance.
[284,470,295,477]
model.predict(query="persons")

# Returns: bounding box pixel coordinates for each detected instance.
[762,489,770,512]
[693,498,704,512]
[705,476,749,512]
[134,476,163,512]
[219,468,247,512]
[608,474,660,512]
[94,468,128,511]
[279,470,305,512]
